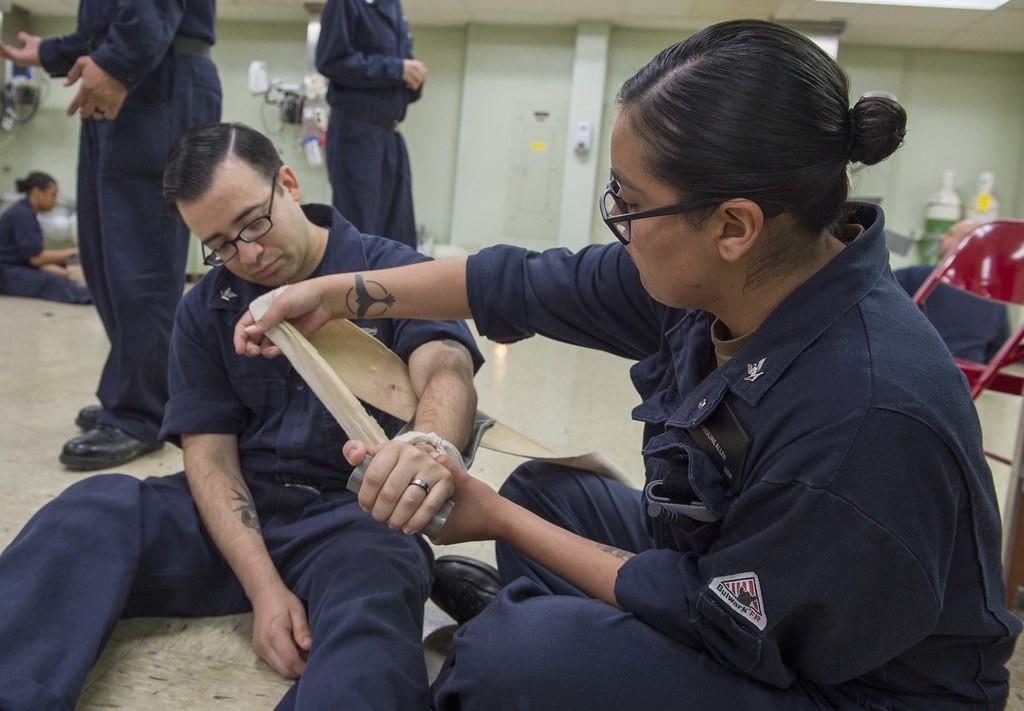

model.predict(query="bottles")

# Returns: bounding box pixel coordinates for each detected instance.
[967,171,999,227]
[919,170,961,268]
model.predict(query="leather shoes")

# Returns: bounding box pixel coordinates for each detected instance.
[59,424,164,471]
[74,405,104,431]
[429,555,501,624]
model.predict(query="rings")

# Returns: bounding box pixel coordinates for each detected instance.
[96,108,105,114]
[410,479,431,494]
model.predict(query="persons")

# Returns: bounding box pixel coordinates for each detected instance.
[230,19,1024,711]
[0,0,224,470]
[0,124,484,711]
[0,172,96,305]
[315,0,426,248]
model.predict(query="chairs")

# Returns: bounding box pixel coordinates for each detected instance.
[913,220,1024,467]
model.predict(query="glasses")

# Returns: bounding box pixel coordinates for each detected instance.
[599,168,786,245]
[201,171,278,268]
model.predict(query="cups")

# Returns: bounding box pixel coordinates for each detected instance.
[301,137,322,168]
